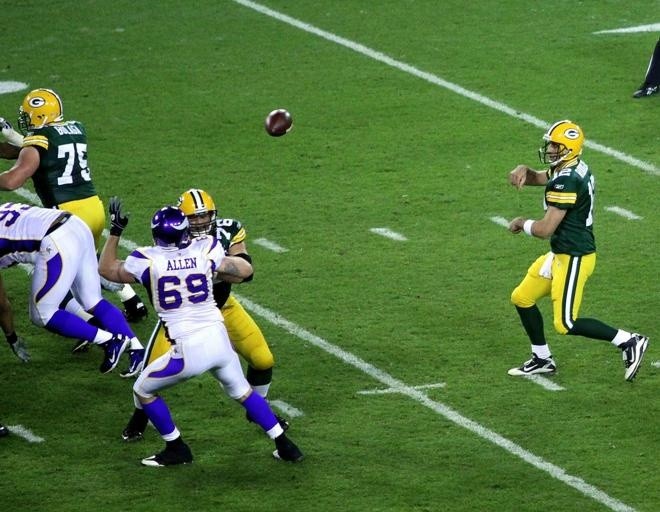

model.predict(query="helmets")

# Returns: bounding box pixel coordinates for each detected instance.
[538,119,584,168]
[18,87,65,136]
[149,205,192,247]
[176,187,217,238]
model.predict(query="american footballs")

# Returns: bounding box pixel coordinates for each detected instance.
[265,108,293,137]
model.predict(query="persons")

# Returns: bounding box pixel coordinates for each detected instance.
[0,116,24,143]
[121,189,290,443]
[508,121,649,381]
[633,39,660,96]
[0,202,147,378]
[0,87,147,354]
[0,423,9,437]
[99,196,304,467]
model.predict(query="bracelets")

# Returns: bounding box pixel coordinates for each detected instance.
[522,217,535,236]
[6,330,17,344]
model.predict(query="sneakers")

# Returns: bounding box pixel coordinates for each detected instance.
[139,442,194,468]
[71,326,109,356]
[632,83,659,98]
[120,408,149,443]
[122,297,149,323]
[507,351,558,377]
[616,331,650,382]
[118,334,147,380]
[270,442,304,463]
[244,407,290,431]
[95,331,132,375]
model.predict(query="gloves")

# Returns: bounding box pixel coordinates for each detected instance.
[108,195,131,238]
[9,335,32,363]
